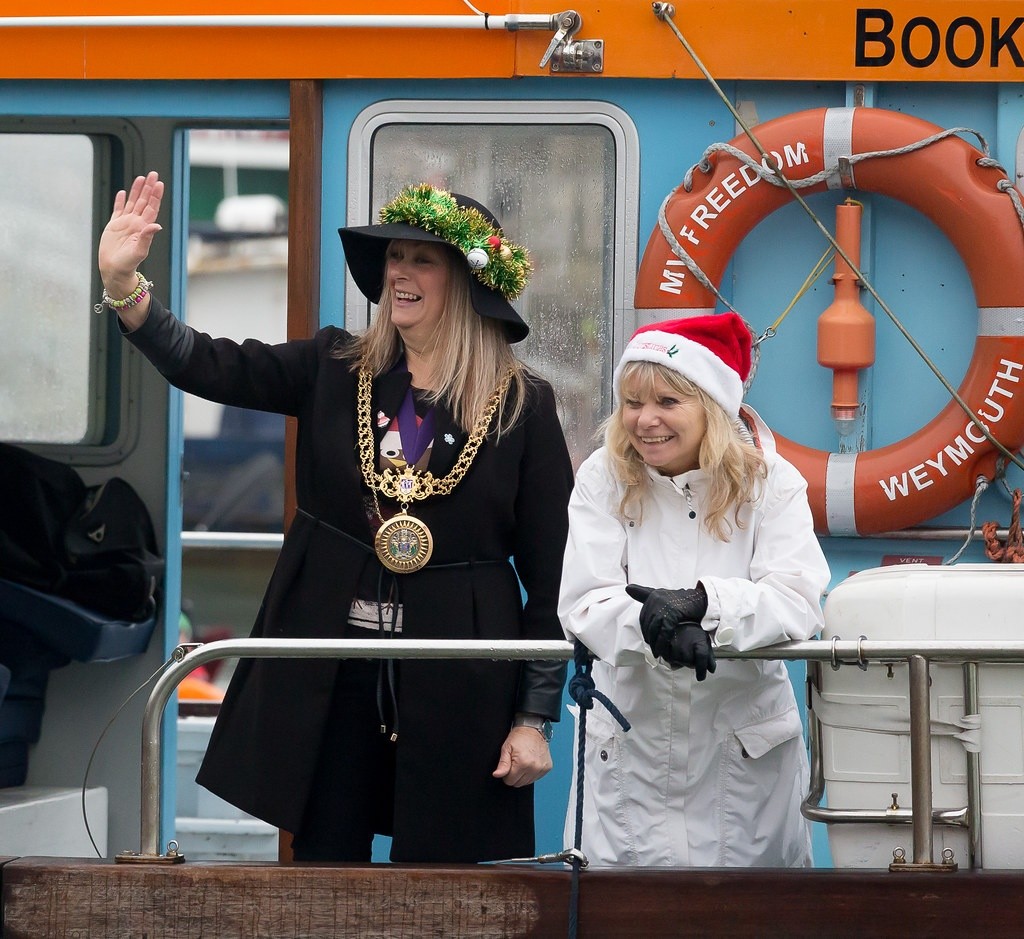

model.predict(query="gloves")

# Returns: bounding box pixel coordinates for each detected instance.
[625,584,707,658]
[660,620,716,681]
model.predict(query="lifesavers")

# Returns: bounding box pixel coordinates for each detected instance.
[631,100,1024,544]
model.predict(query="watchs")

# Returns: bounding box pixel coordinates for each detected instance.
[514,716,553,742]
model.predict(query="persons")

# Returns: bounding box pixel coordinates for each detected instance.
[98,170,573,865]
[557,311,832,867]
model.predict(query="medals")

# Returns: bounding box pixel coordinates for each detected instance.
[375,517,433,572]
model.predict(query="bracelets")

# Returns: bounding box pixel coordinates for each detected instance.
[94,272,153,314]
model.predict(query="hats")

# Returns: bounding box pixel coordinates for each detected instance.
[613,312,752,422]
[338,193,530,345]
